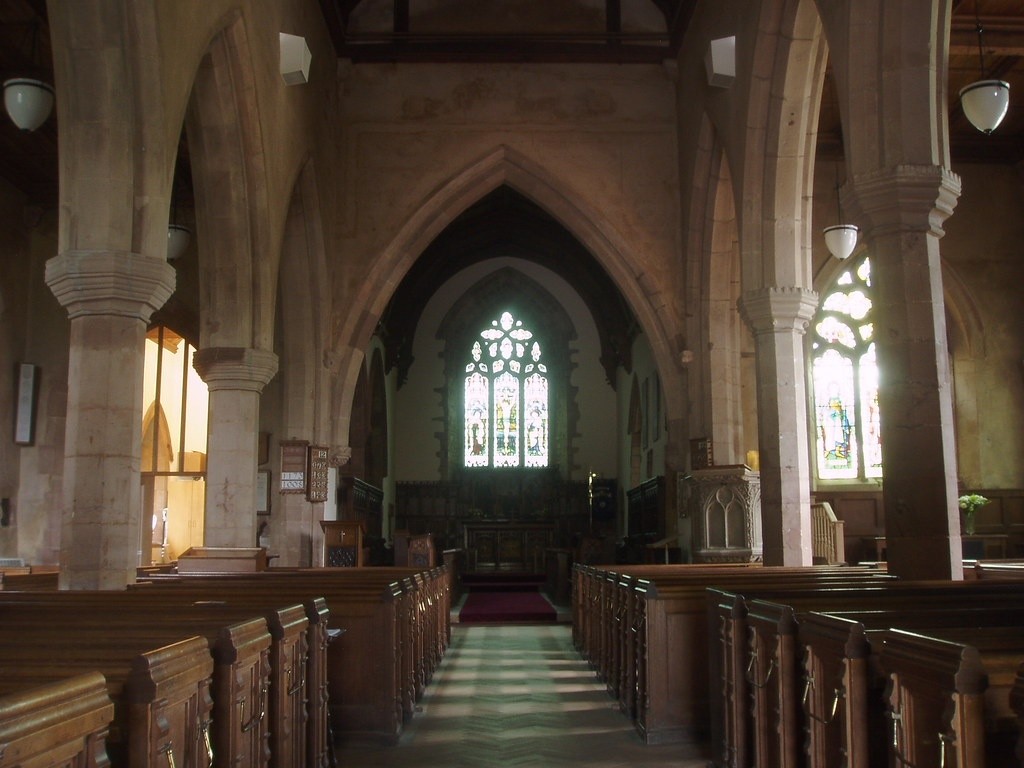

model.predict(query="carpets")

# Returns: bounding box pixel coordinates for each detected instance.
[459,593,557,622]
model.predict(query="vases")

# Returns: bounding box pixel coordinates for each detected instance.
[965,510,977,535]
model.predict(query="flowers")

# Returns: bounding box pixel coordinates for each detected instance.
[959,493,992,511]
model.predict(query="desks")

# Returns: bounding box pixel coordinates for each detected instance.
[860,535,1009,561]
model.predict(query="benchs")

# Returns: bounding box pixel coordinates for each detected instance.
[0,565,455,768]
[569,560,1024,768]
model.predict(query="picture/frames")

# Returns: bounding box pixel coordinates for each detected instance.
[256,470,271,514]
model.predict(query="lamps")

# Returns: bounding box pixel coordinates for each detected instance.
[823,160,858,261]
[2,17,55,134]
[168,166,193,263]
[959,0,1012,135]
